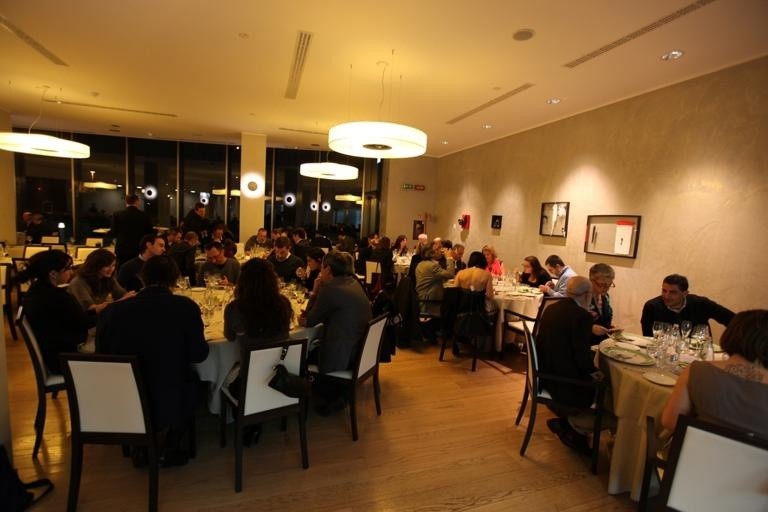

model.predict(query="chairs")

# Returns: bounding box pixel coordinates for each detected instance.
[221,338,309,492]
[1,235,368,338]
[514,316,612,474]
[14,304,67,462]
[364,240,595,372]
[59,351,216,510]
[639,414,768,509]
[306,312,391,442]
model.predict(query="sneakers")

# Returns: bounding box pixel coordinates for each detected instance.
[545,416,575,449]
[572,432,594,456]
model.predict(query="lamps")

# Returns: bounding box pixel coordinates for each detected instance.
[327,61,428,168]
[1,85,92,160]
[299,160,360,182]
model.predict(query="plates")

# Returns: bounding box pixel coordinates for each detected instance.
[643,372,677,386]
[597,328,654,366]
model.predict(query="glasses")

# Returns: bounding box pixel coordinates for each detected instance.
[593,277,616,291]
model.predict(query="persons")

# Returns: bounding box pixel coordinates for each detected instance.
[536,274,618,453]
[660,308,768,442]
[585,262,624,341]
[639,273,741,347]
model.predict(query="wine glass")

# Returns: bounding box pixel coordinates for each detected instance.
[492,268,530,297]
[174,274,305,330]
[196,241,268,261]
[392,247,415,264]
[648,319,711,375]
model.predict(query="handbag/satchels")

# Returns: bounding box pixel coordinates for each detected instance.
[267,363,310,400]
[451,310,494,359]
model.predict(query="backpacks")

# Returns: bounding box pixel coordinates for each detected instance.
[0,443,55,512]
[378,288,403,335]
